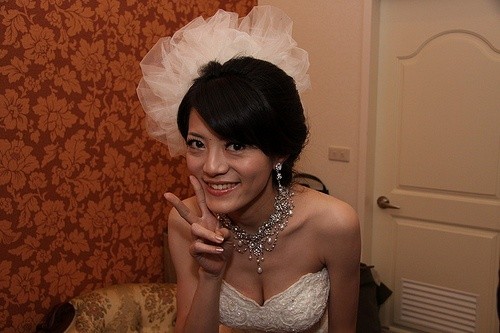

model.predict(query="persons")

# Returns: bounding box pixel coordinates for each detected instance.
[136,3,360,333]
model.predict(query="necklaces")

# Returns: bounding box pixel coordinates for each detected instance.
[214,184,296,274]
